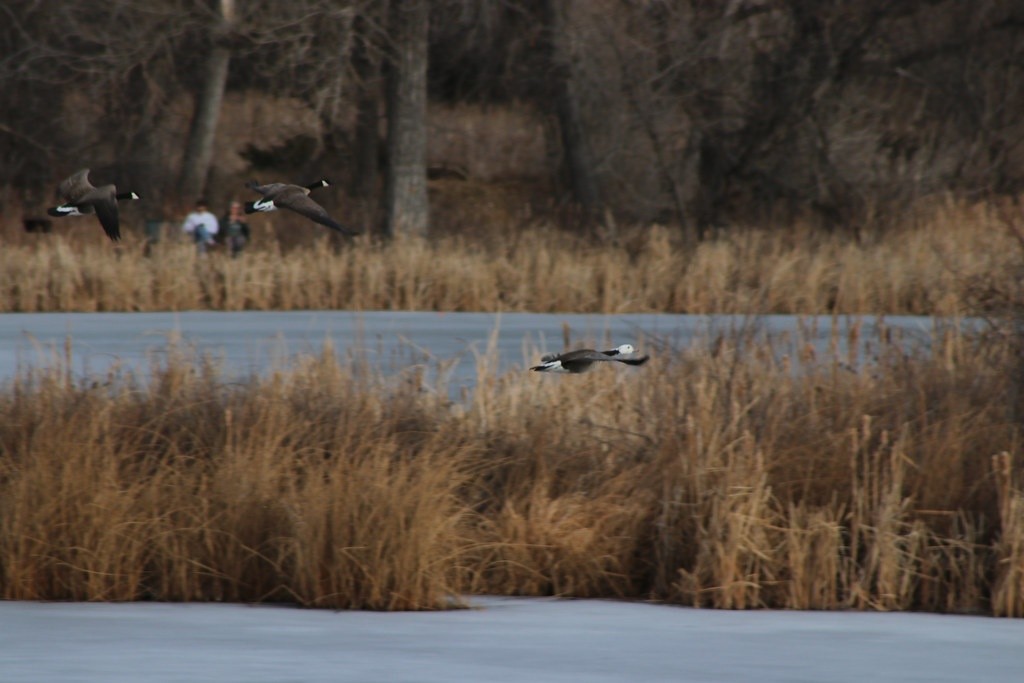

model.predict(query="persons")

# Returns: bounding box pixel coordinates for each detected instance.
[179,200,250,258]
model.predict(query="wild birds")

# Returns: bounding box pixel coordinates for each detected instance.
[46,168,141,243]
[244,173,356,230]
[531,344,651,374]
